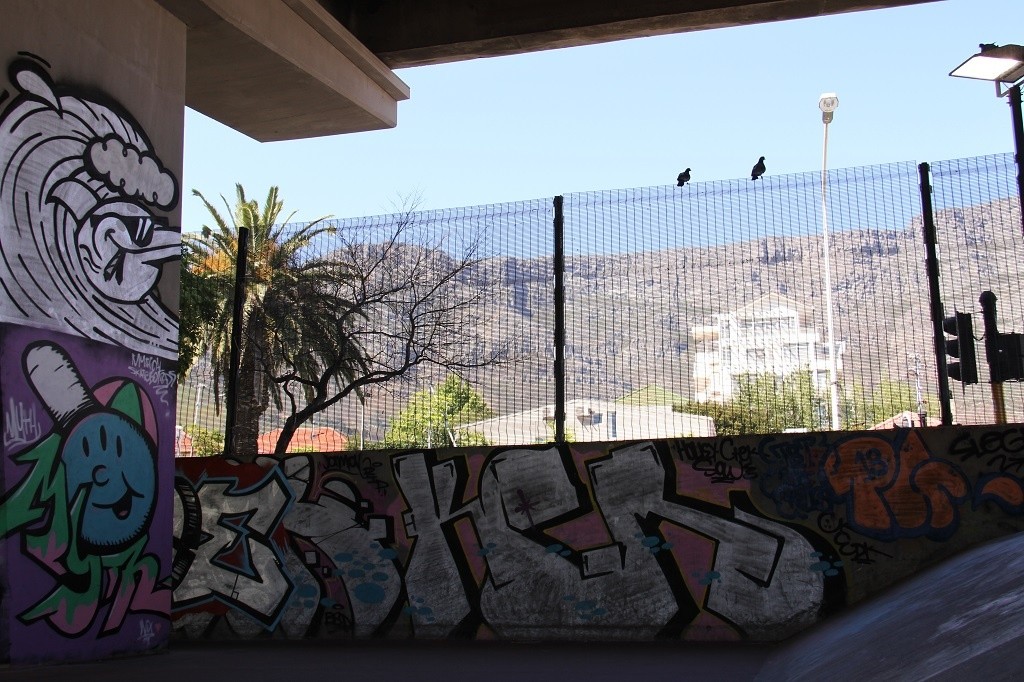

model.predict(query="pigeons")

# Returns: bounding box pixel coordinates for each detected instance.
[750,155,767,181]
[676,167,692,187]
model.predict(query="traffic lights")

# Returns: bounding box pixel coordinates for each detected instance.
[944,310,977,383]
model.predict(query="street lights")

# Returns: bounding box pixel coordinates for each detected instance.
[947,41,1023,232]
[816,92,841,430]
